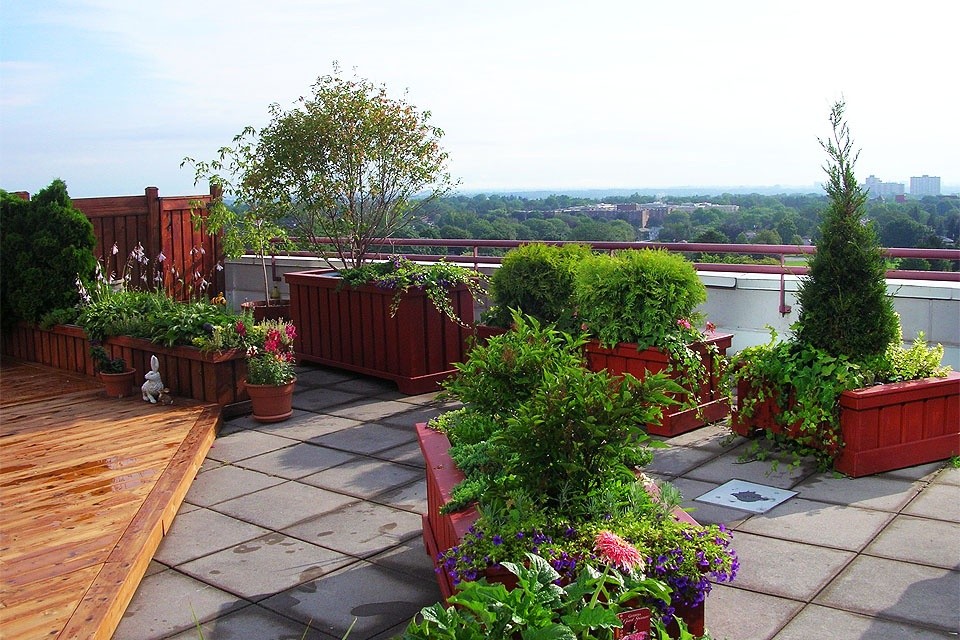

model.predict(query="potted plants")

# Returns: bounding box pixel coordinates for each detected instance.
[415,306,705,640]
[181,125,290,323]
[724,98,960,479]
[473,242,594,373]
[576,249,733,437]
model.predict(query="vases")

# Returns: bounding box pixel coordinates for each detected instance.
[244,376,296,423]
[287,267,479,394]
[99,368,136,397]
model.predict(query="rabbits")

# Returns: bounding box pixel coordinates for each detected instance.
[140,354,164,403]
[157,388,173,405]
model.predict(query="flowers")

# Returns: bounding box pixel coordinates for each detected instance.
[89,337,127,377]
[341,257,494,333]
[234,317,299,385]
